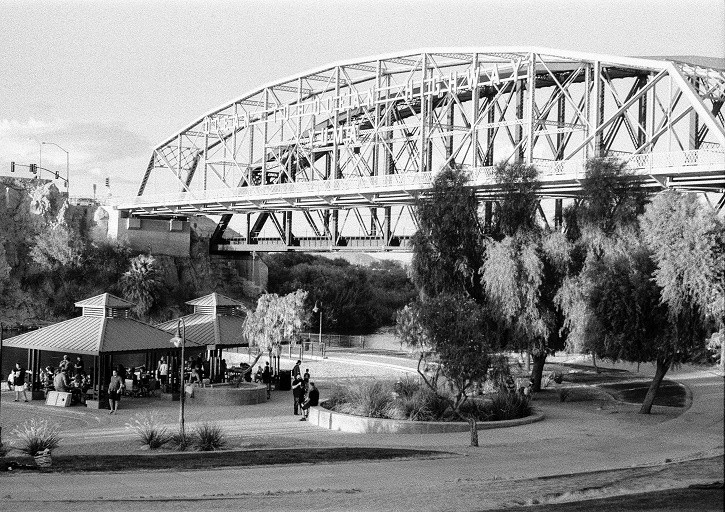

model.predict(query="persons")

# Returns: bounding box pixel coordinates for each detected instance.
[221,359,271,390]
[108,355,204,416]
[292,360,320,421]
[7,355,92,405]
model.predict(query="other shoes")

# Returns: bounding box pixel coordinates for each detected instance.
[109,411,113,414]
[13,399,19,402]
[25,400,28,402]
[294,413,299,415]
[305,414,308,417]
[299,418,306,421]
[114,411,117,415]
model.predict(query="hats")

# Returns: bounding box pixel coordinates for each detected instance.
[297,375,301,379]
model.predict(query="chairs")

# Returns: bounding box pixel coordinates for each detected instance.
[125,374,210,399]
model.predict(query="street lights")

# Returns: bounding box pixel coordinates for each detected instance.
[169,318,187,442]
[311,299,323,342]
